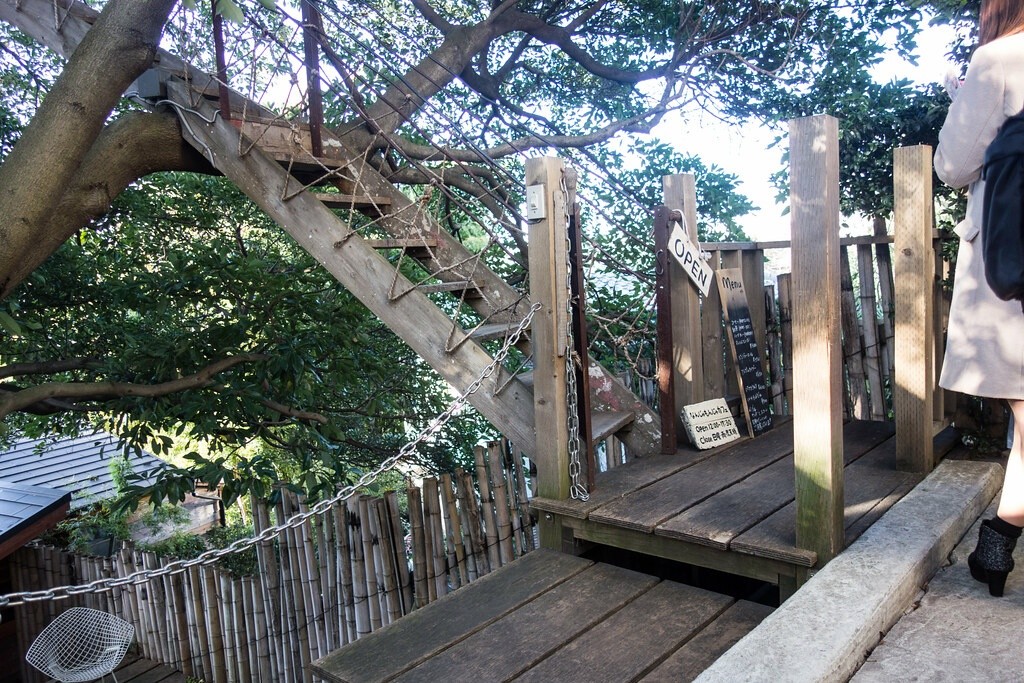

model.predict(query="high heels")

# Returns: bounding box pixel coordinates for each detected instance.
[968,519,1016,597]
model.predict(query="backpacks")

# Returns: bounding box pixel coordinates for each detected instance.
[980,109,1024,313]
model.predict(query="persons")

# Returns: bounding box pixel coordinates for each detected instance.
[933,0,1024,596]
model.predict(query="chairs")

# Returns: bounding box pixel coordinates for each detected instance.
[26,606,135,683]
